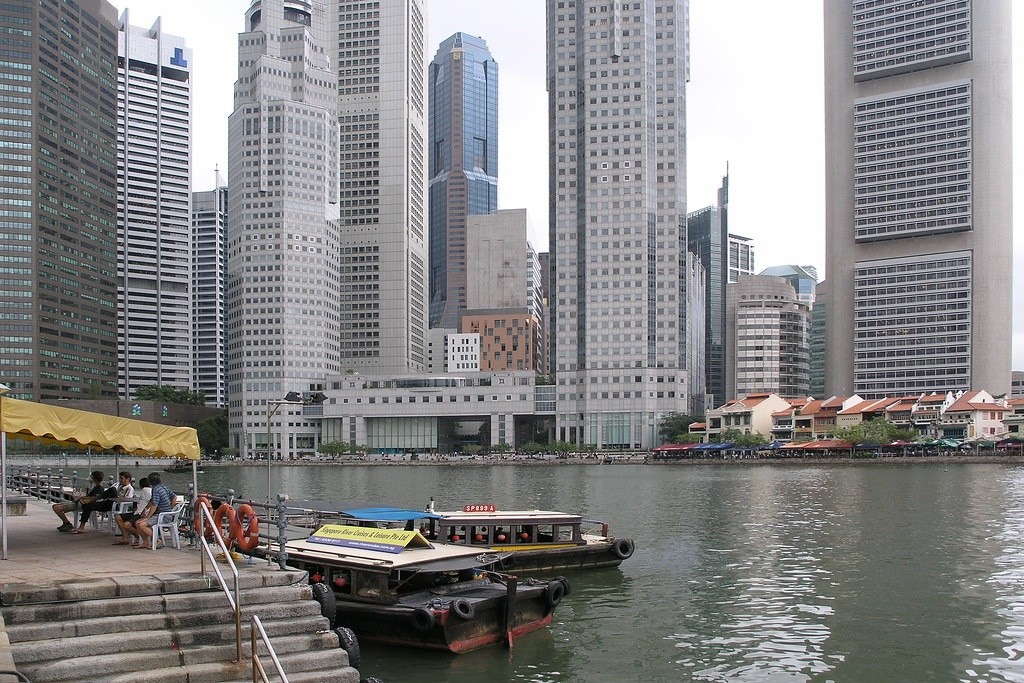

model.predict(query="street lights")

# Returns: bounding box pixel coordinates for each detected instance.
[266,390,329,566]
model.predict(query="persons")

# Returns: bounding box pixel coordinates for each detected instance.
[111,477,152,546]
[133,472,177,550]
[68,472,134,534]
[52,472,105,532]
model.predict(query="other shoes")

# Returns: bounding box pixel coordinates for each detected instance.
[56,521,74,532]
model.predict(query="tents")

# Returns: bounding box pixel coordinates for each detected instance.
[651,432,1024,458]
[0,395,201,560]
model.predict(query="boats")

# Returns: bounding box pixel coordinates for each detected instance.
[164,455,206,474]
[217,521,572,656]
[256,496,636,577]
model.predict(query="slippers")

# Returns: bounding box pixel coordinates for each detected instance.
[133,545,147,549]
[148,545,164,549]
[71,530,84,534]
[79,499,95,505]
[111,541,129,545]
[131,542,140,546]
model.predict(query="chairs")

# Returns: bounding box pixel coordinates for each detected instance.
[150,502,186,550]
[73,496,144,545]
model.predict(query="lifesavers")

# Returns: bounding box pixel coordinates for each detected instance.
[546,581,564,608]
[552,575,571,597]
[312,582,336,630]
[626,538,635,552]
[334,627,361,672]
[215,503,236,550]
[613,538,634,560]
[193,496,214,538]
[449,598,475,622]
[234,504,259,553]
[411,607,435,633]
